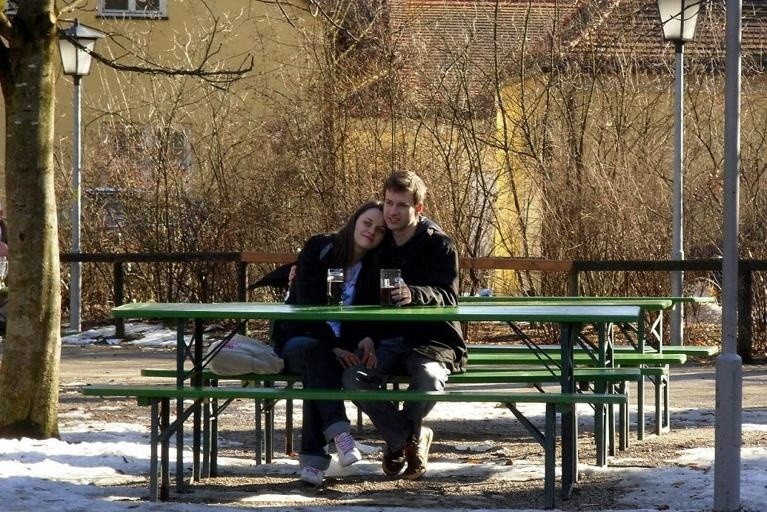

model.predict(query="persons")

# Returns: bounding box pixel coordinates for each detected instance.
[341,169,468,480]
[270,199,387,483]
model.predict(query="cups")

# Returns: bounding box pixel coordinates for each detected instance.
[327,269,343,307]
[380,268,403,308]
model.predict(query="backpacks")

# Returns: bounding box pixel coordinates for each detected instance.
[208,332,285,376]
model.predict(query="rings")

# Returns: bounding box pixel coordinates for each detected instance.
[368,351,374,357]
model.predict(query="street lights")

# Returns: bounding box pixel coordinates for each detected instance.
[656,0,702,346]
[54,18,107,333]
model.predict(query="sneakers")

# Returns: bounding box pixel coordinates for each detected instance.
[402,424,434,480]
[381,433,406,476]
[300,465,324,486]
[333,431,362,467]
